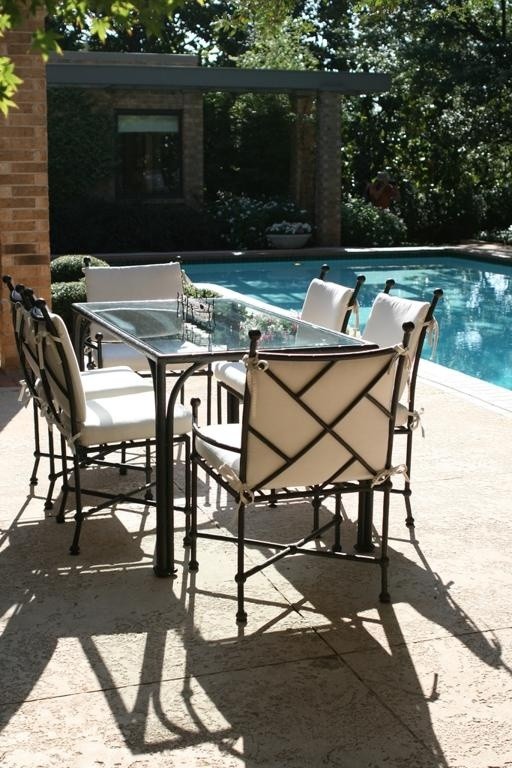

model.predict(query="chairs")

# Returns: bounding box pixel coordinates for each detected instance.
[195,319,416,622]
[71,255,188,473]
[321,279,443,552]
[300,263,366,335]
[0,280,193,553]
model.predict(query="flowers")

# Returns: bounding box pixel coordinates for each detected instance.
[265,220,313,234]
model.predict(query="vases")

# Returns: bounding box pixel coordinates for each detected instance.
[266,234,313,249]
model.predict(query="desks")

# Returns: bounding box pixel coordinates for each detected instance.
[61,292,380,577]
